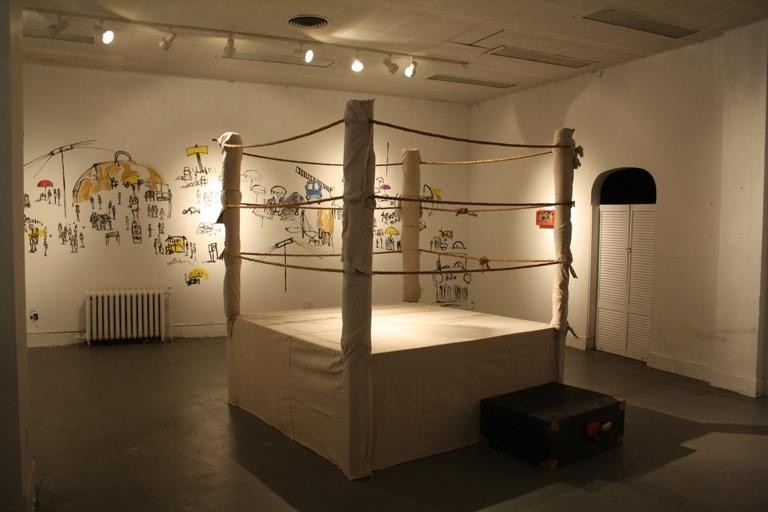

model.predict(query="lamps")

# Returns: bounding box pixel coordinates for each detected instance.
[44,12,416,78]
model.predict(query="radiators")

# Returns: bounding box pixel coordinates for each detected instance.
[85,285,173,343]
